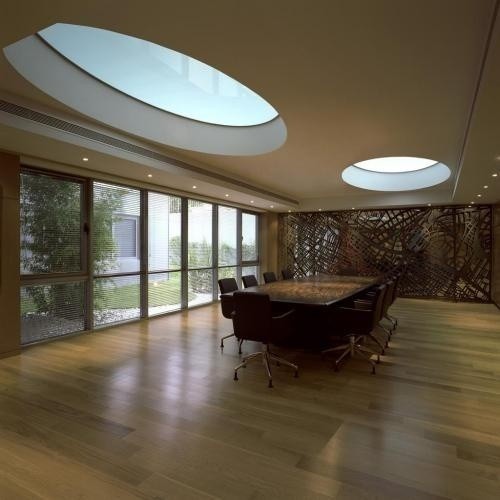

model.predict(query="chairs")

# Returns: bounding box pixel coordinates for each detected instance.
[217,269,401,388]
